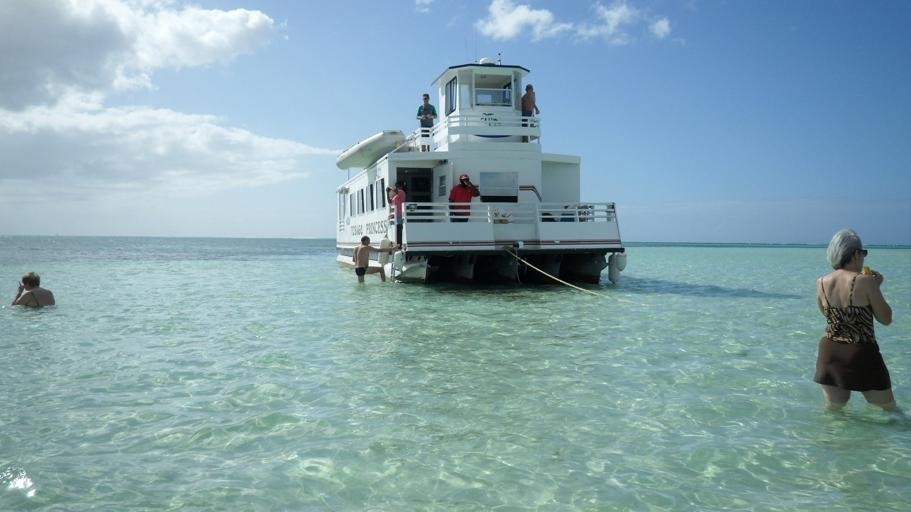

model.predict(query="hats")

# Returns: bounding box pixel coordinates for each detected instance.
[460,175,469,180]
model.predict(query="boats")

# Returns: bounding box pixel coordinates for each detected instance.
[334,52,628,290]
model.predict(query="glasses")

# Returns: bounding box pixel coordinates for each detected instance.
[853,249,868,257]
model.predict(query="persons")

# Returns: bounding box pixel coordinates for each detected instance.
[351,235,401,284]
[811,226,899,412]
[385,178,408,245]
[448,173,480,222]
[416,93,438,152]
[522,84,541,143]
[9,272,56,307]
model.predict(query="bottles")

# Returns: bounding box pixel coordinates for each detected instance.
[497,52,502,65]
